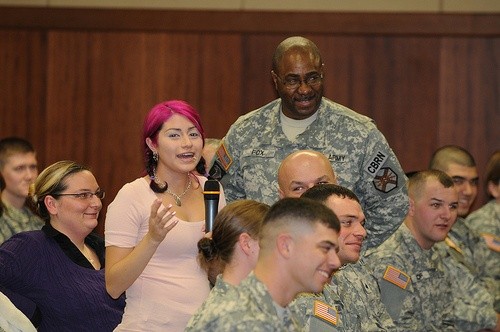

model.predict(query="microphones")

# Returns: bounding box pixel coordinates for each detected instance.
[203,180,220,234]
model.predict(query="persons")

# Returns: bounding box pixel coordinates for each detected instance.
[209,35,500,332]
[0,291,38,332]
[193,197,342,332]
[298,183,367,332]
[0,158,126,332]
[1,134,46,245]
[103,101,228,332]
[184,200,271,332]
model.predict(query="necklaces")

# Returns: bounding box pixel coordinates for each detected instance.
[154,170,192,207]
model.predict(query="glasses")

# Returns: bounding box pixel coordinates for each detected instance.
[46,190,105,200]
[273,69,323,89]
